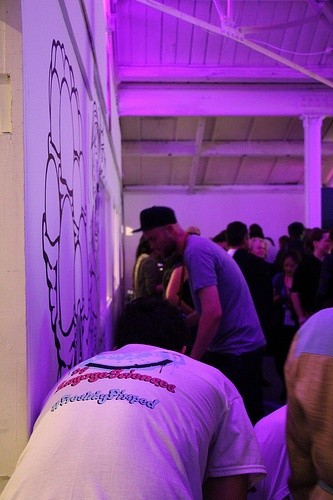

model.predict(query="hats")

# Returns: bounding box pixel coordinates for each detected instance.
[131,205,178,233]
[187,226,201,236]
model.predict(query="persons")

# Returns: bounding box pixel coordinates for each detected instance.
[131,205,267,426]
[248,404,294,500]
[283,307,333,500]
[0,296,268,500]
[113,221,333,405]
